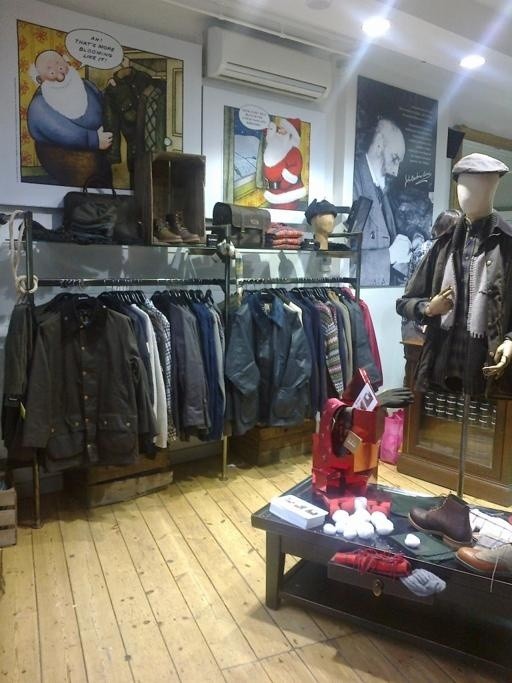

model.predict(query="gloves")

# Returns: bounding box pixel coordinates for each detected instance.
[377,387,415,408]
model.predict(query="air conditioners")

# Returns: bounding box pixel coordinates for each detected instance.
[206,25,332,104]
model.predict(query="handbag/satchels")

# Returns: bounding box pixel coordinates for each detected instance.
[64,174,145,243]
[380,409,406,465]
[212,202,271,248]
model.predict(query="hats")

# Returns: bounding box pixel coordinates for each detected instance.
[452,153,509,181]
[305,199,337,225]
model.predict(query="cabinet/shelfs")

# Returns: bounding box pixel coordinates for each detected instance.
[251,471,512,679]
[400,341,511,506]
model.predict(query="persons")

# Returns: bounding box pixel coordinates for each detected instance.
[396,152,510,399]
[353,119,410,287]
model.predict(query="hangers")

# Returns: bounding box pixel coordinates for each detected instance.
[57,276,222,314]
[238,276,363,310]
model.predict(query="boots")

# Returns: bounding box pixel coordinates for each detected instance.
[408,493,474,550]
[153,217,182,243]
[168,214,201,242]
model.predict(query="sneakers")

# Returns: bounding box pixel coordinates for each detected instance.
[455,542,512,579]
